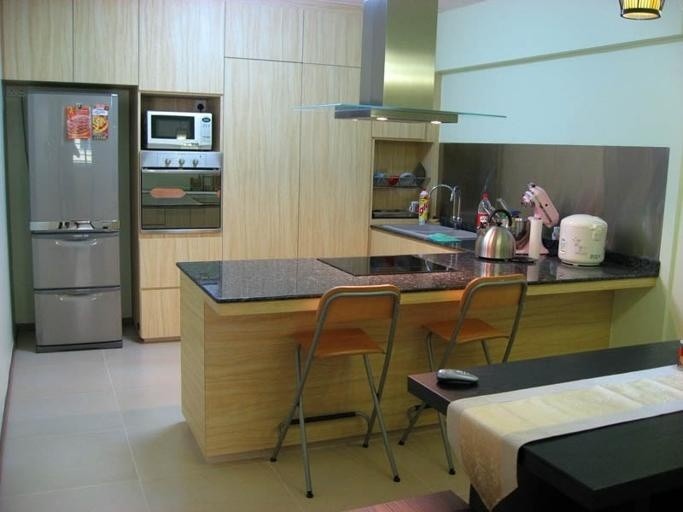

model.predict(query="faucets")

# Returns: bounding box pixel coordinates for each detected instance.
[428,183,457,222]
[450,185,464,222]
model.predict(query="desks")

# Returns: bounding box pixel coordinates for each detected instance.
[407,339,683,512]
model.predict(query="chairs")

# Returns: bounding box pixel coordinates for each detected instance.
[398,271,527,475]
[270,285,400,499]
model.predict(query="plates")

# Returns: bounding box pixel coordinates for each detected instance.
[399,172,416,186]
[511,257,535,263]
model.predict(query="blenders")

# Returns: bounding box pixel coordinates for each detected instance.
[502,181,560,255]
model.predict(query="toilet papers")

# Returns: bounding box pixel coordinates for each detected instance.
[527,216,543,260]
[526,265,540,281]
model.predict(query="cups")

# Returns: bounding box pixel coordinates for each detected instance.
[409,201,417,212]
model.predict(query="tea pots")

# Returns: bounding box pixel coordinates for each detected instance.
[476,208,517,260]
[477,262,524,278]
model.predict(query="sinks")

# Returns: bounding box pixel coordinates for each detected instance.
[410,229,478,249]
[370,220,454,233]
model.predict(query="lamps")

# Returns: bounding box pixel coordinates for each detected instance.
[618,0,664,19]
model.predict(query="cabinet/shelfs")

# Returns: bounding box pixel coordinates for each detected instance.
[74,0,140,86]
[219,57,373,262]
[224,0,365,67]
[130,230,223,343]
[138,0,226,97]
[0,0,73,89]
[373,122,440,223]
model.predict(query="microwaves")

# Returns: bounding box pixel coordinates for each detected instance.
[142,111,213,151]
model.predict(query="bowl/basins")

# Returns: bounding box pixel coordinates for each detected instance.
[388,176,399,184]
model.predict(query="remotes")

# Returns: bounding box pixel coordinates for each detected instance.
[437,369,479,382]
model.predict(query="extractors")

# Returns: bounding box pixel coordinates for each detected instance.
[292,2,512,124]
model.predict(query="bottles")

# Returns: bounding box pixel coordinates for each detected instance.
[527,264,541,284]
[530,217,542,260]
[373,170,386,186]
[475,193,491,228]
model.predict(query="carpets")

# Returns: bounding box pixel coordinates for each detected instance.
[343,489,470,512]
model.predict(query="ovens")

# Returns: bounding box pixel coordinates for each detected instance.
[138,151,221,228]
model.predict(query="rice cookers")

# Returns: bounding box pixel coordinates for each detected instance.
[555,266,605,283]
[558,213,609,268]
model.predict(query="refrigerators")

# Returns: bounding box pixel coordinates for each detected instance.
[19,91,123,348]
